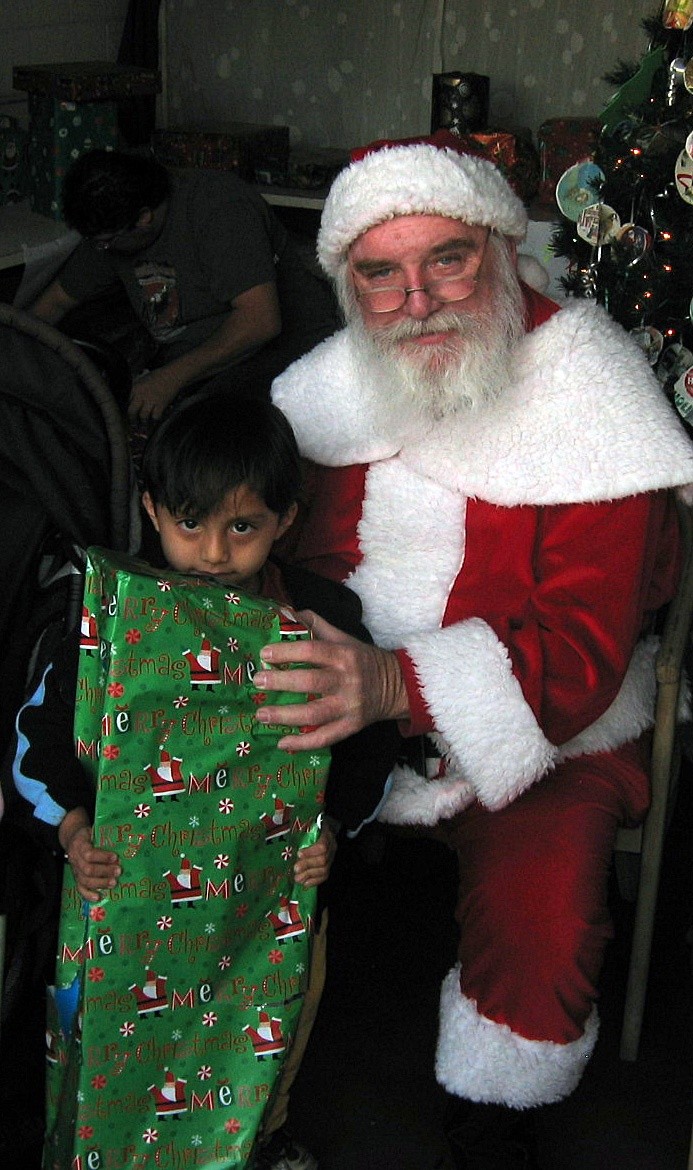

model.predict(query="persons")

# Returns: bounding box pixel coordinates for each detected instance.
[10,140,409,1170]
[262,129,691,1170]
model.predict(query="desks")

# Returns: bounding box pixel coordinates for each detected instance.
[0,207,83,270]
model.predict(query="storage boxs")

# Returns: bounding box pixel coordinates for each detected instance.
[467,117,601,207]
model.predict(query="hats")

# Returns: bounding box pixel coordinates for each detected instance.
[315,131,529,277]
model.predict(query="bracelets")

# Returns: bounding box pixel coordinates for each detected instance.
[66,823,85,860]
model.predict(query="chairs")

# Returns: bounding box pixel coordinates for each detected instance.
[0,302,132,631]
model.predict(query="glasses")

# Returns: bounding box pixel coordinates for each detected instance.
[79,212,139,250]
[348,226,488,313]
